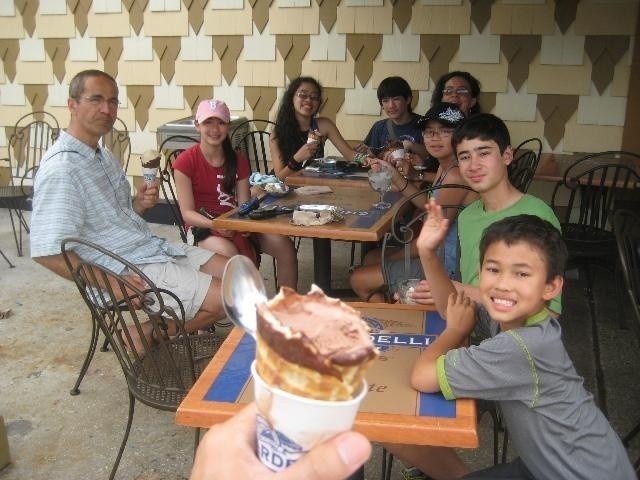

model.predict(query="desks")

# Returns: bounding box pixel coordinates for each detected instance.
[213,183,413,297]
[284,156,423,265]
[175,301,480,479]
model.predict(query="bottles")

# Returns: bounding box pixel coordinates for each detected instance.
[199,208,220,224]
[397,278,421,306]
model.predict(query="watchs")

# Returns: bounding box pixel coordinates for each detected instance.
[430,71,481,119]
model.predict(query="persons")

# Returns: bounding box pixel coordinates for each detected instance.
[408,196,639,480]
[172,99,297,297]
[28,70,234,358]
[268,78,376,180]
[187,399,374,480]
[352,100,480,303]
[355,77,427,157]
[413,112,563,407]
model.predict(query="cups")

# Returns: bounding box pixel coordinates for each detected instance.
[250,356,368,471]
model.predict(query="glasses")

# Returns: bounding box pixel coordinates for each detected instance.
[296,91,320,102]
[442,85,472,97]
[75,94,121,109]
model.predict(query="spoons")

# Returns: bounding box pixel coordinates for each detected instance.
[220,253,267,343]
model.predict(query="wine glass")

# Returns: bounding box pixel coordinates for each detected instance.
[367,166,393,209]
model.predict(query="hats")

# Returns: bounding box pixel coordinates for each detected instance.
[193,99,231,126]
[417,102,465,128]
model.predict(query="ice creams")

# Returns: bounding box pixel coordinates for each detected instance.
[301,129,322,167]
[250,280,379,472]
[386,140,407,181]
[140,148,161,189]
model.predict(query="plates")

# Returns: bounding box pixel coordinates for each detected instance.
[299,204,338,211]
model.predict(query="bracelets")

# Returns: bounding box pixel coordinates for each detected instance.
[288,157,304,171]
[416,168,426,181]
[398,177,410,194]
[352,148,370,164]
